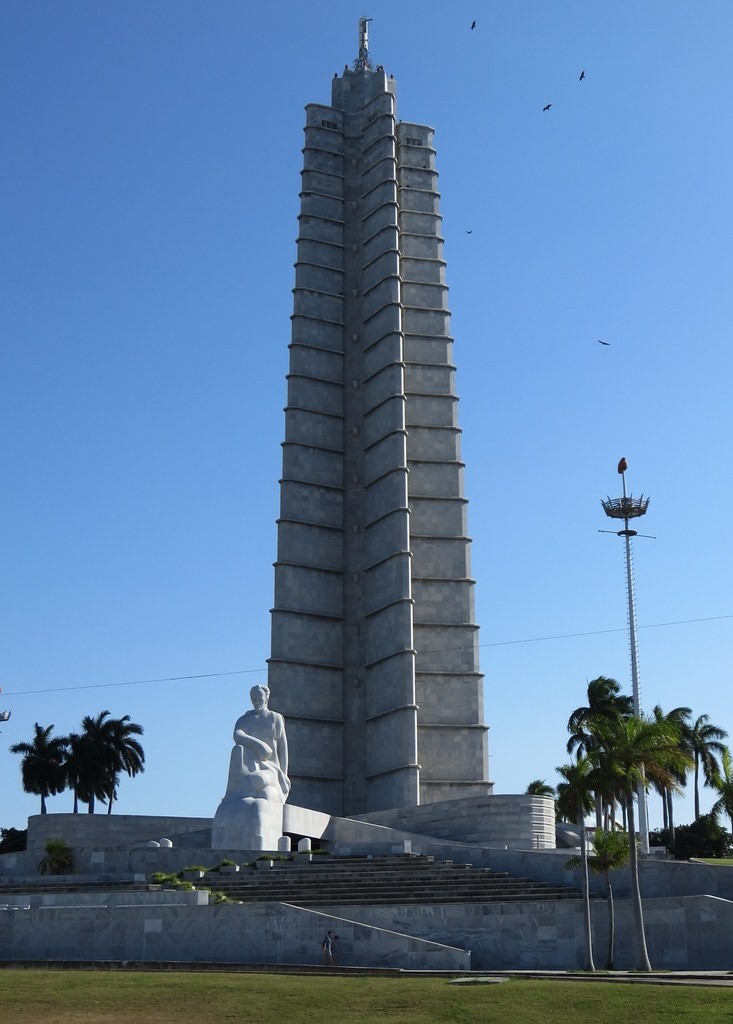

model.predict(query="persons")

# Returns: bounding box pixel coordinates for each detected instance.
[233,685,292,794]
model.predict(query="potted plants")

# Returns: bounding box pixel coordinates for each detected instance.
[39,839,73,875]
[184,865,208,878]
[255,855,274,869]
[293,849,330,862]
[148,872,243,905]
[218,859,241,873]
